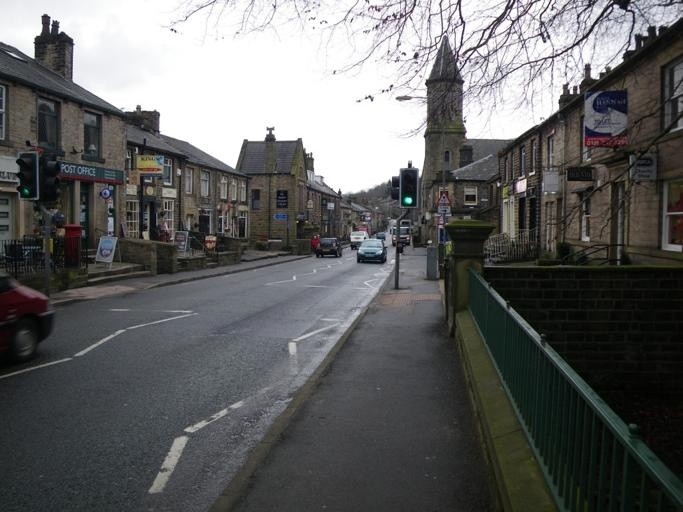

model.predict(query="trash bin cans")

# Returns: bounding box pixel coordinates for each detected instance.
[426,246,439,281]
[64,224,83,263]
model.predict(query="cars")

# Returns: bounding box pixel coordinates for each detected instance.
[315,237,341,257]
[0,267,56,360]
[348,216,411,250]
[355,238,388,263]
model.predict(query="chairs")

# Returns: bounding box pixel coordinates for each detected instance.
[33,250,56,273]
[4,244,31,280]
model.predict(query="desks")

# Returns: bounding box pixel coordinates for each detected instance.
[21,246,41,272]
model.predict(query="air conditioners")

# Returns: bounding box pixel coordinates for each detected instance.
[127,148,131,159]
[221,176,228,183]
[241,180,246,188]
[231,178,238,186]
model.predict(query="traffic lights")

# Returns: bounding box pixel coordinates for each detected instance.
[399,168,416,208]
[391,176,399,200]
[14,152,38,198]
[39,154,61,204]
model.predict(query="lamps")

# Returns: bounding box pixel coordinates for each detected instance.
[88,144,96,155]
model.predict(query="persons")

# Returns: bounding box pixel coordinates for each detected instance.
[310,234,323,258]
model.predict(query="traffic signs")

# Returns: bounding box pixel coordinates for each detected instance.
[272,213,287,219]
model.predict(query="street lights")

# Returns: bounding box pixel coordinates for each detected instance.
[392,89,447,263]
